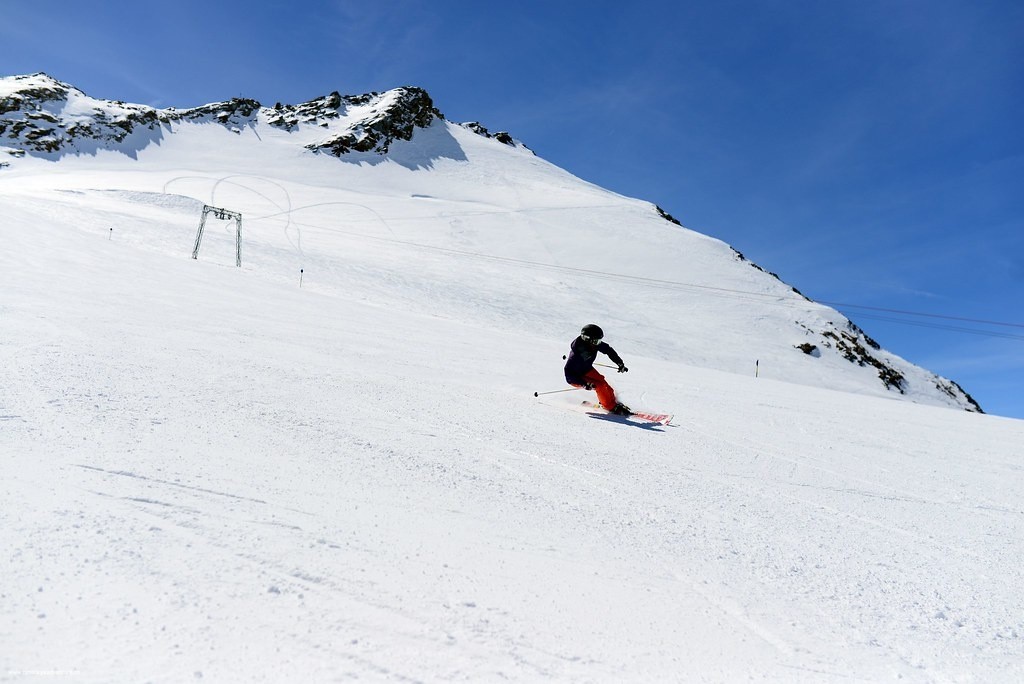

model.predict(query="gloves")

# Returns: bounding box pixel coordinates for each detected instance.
[618,366,626,373]
[585,383,594,390]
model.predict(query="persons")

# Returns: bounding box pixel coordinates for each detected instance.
[564,324,634,418]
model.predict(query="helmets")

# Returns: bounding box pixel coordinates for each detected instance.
[581,325,603,344]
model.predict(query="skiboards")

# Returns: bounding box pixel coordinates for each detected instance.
[581,400,675,427]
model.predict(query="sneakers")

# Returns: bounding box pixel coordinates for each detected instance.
[610,401,634,417]
